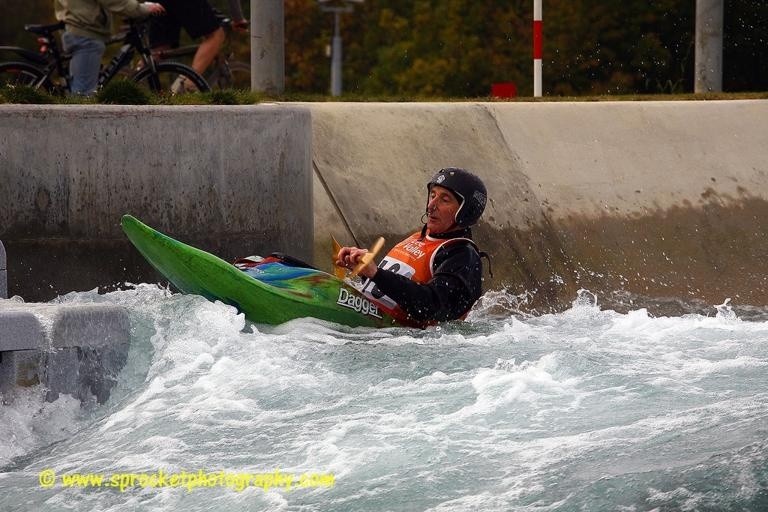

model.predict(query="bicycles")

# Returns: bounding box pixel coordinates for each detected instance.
[111,18,254,94]
[1,3,218,97]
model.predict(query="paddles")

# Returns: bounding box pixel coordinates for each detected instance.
[330,233,386,280]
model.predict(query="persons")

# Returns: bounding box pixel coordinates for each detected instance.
[54,0,165,97]
[138,0,226,95]
[334,168,488,327]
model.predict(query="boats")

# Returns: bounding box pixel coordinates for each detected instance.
[101,203,392,339]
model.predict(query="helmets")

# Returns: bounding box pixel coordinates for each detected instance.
[424,166,489,228]
